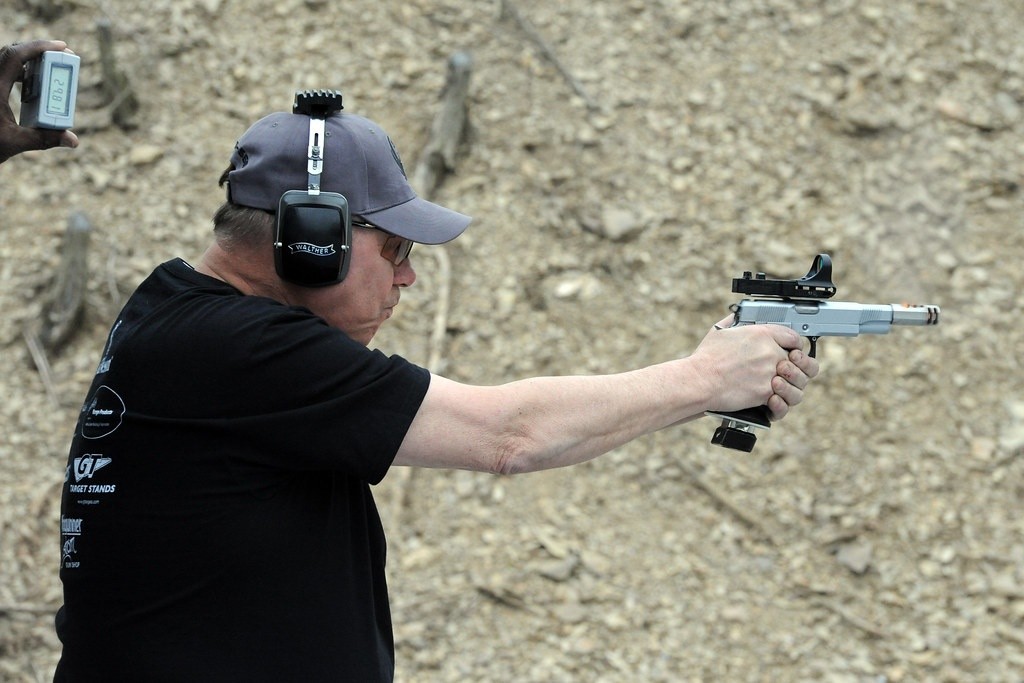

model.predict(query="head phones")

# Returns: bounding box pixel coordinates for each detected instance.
[274,90,353,289]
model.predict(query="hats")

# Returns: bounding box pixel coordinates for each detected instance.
[226,112,473,245]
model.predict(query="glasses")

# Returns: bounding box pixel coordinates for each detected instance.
[351,221,413,266]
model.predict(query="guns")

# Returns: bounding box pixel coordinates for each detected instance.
[701,252,943,453]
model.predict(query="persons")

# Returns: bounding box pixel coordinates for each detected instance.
[0,38,78,160]
[52,115,819,683]
